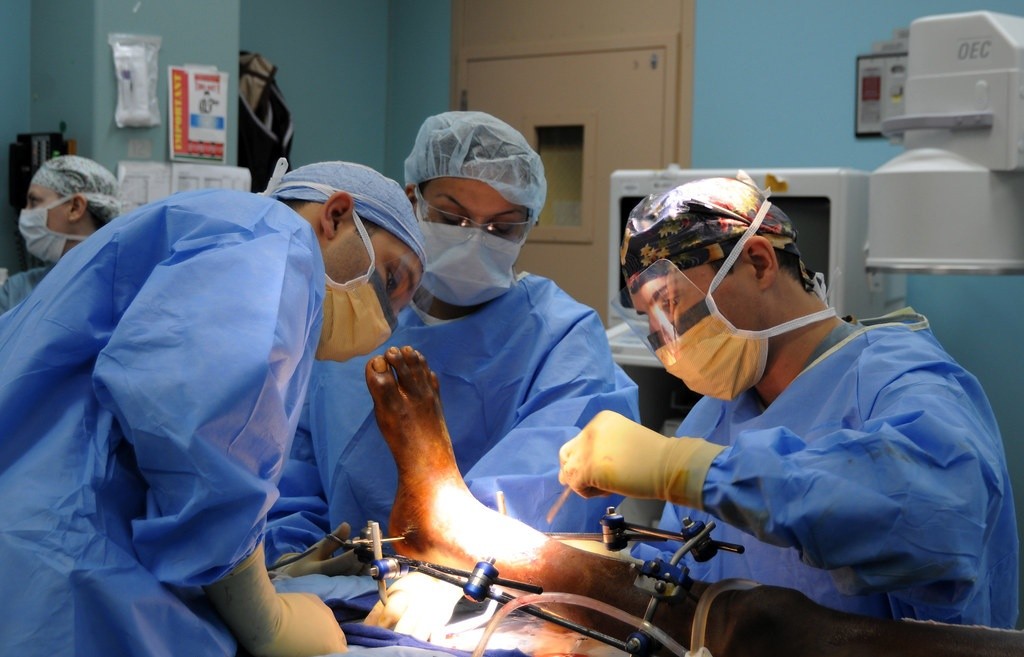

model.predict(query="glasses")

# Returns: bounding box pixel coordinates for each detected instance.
[415,183,532,245]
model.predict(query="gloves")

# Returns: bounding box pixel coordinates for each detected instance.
[199,539,348,657]
[273,521,372,575]
[558,409,728,513]
[364,570,465,647]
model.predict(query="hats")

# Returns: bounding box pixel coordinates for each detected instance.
[618,174,812,292]
[273,162,427,285]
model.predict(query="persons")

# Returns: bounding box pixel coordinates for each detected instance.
[0,160,426,657]
[262,111,642,579]
[557,177,1020,627]
[0,155,123,311]
[366,345,1024,657]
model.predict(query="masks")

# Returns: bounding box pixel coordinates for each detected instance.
[18,195,74,266]
[315,210,398,362]
[646,246,776,401]
[413,186,529,307]
